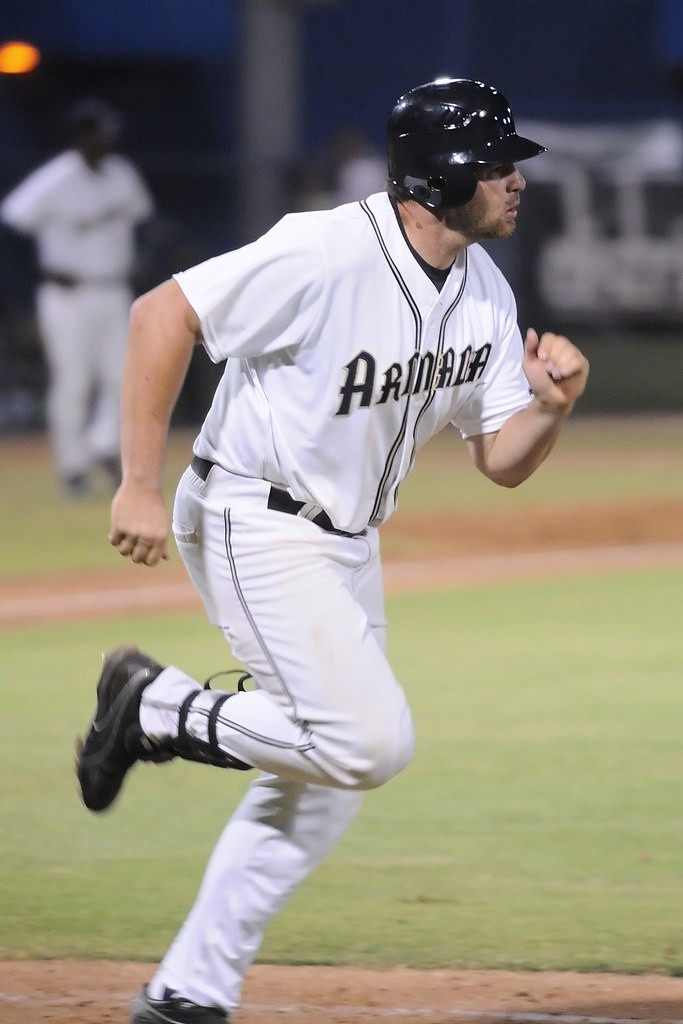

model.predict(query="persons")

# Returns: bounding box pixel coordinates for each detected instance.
[72,79,591,1024]
[4,103,156,498]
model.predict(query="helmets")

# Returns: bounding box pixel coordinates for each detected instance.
[387,79,548,210]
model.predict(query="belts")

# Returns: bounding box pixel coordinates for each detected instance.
[191,456,368,538]
[39,270,79,291]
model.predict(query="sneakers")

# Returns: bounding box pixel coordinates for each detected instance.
[77,646,165,811]
[129,982,232,1024]
